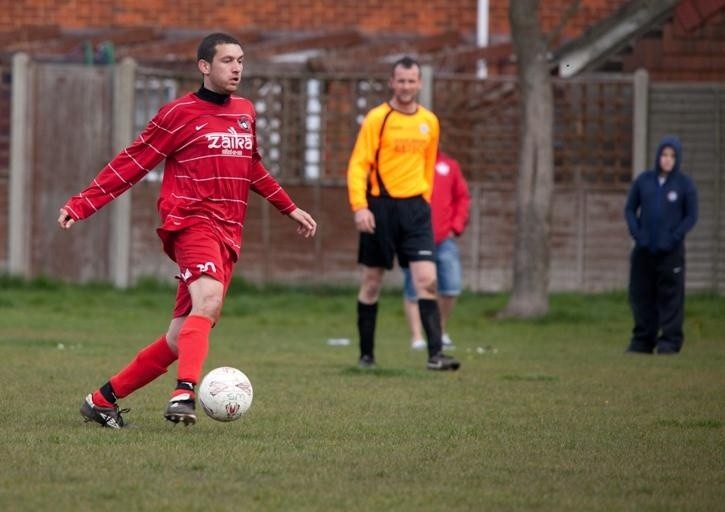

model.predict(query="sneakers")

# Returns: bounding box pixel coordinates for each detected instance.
[412,339,427,352]
[164,393,197,426]
[440,332,454,348]
[79,393,131,431]
[427,351,460,370]
[359,355,376,370]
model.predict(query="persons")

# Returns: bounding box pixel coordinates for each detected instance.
[57,33,318,432]
[400,149,472,353]
[345,55,463,371]
[619,136,701,355]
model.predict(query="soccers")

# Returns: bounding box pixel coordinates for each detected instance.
[198,365,253,422]
